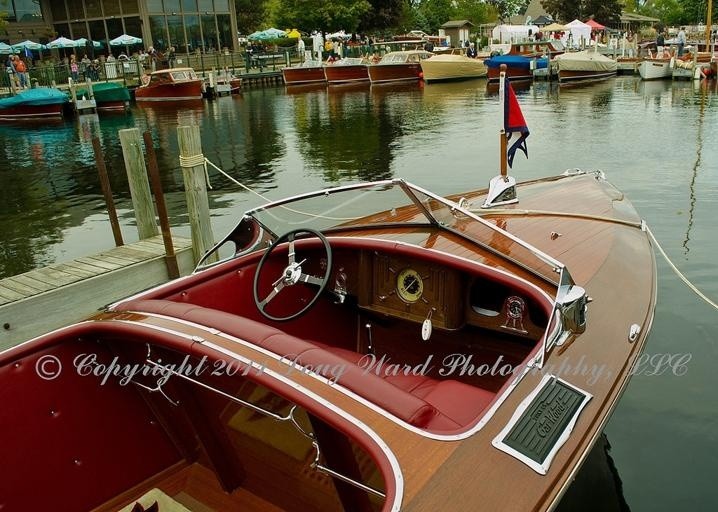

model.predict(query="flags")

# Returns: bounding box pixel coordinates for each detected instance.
[503,78,529,169]
[0,34,143,59]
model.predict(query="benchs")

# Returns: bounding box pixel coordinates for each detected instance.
[111,299,496,430]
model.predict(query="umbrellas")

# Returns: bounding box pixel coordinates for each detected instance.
[257,28,286,52]
[248,30,264,49]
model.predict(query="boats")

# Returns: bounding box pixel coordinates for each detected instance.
[1,64,659,511]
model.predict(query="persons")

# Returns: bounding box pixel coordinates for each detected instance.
[206,44,216,54]
[192,44,202,66]
[324,36,348,61]
[598,32,606,47]
[221,43,230,67]
[220,66,237,80]
[430,39,470,49]
[142,46,176,70]
[699,18,704,26]
[676,27,686,57]
[13,54,28,90]
[296,36,306,60]
[42,52,139,84]
[656,28,666,59]
[589,32,596,45]
[6,55,19,91]
[424,40,433,59]
[137,50,145,78]
[244,39,278,70]
[528,31,565,42]
[466,42,477,59]
